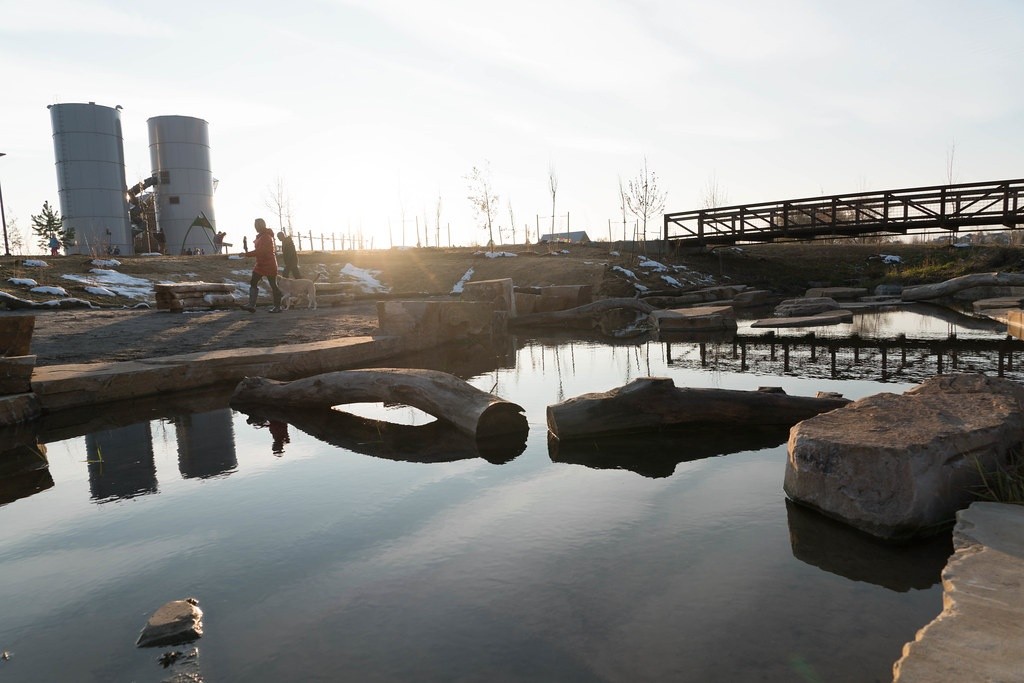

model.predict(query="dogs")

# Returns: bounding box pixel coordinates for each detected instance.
[275,273,321,311]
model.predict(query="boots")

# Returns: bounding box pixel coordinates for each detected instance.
[269,292,281,312]
[240,288,259,313]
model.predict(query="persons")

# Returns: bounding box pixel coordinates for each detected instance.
[213,231,226,254]
[183,248,205,255]
[50,234,59,255]
[243,235,248,253]
[108,245,120,256]
[158,227,166,254]
[277,232,301,279]
[240,218,283,313]
